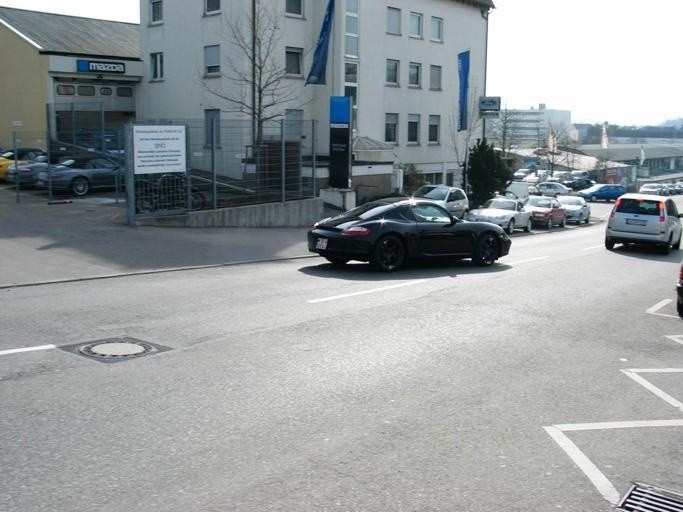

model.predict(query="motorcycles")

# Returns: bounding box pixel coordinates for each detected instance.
[134,168,206,214]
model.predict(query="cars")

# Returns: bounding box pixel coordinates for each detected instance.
[578,184,626,201]
[62,122,126,157]
[413,169,590,235]
[0,147,127,197]
[605,193,683,256]
[639,182,683,196]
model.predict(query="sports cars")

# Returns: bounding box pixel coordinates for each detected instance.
[307,195,511,271]
